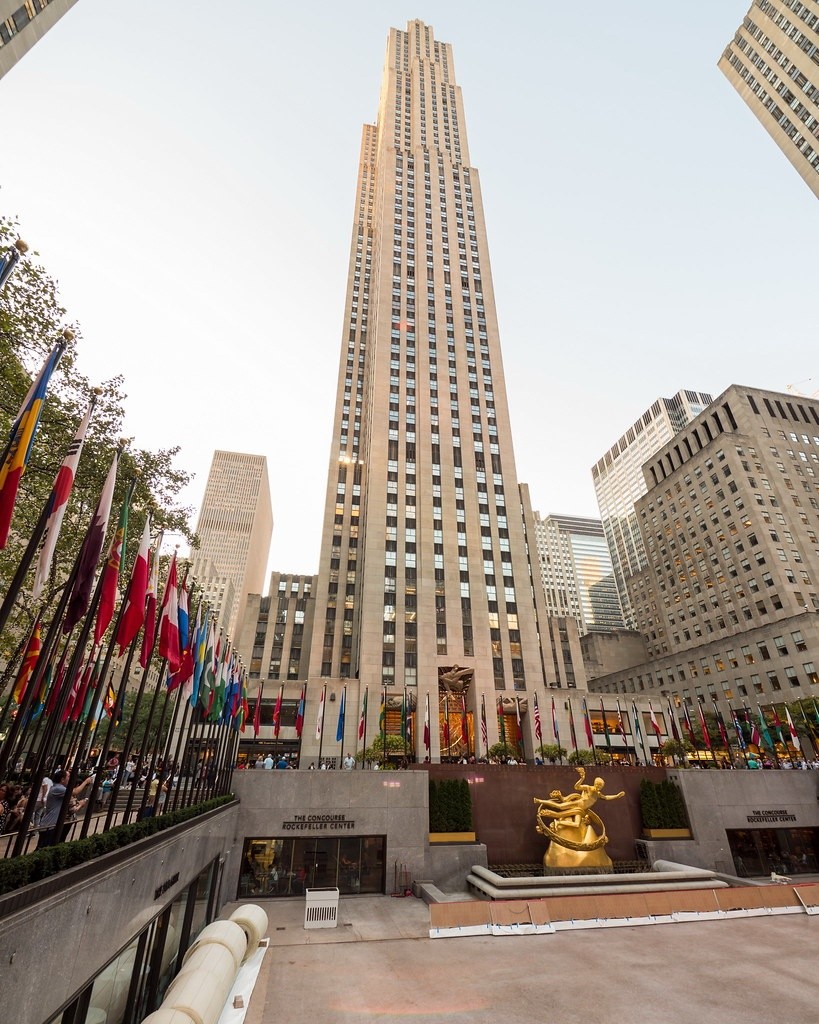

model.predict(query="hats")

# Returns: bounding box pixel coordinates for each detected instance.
[282,757,285,759]
[268,754,272,758]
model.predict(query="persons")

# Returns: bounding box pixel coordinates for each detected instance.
[596,756,670,767]
[533,767,625,833]
[733,849,809,876]
[0,753,217,850]
[692,761,711,769]
[722,757,819,771]
[424,754,585,766]
[237,754,333,770]
[343,753,356,770]
[374,763,380,771]
[401,757,410,769]
[247,852,368,896]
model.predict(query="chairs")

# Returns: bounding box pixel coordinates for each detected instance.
[298,873,307,894]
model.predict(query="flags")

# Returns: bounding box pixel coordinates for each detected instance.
[273,686,283,736]
[316,686,325,739]
[359,689,368,740]
[296,685,305,737]
[336,688,345,742]
[379,686,819,753]
[0,256,249,732]
[253,683,263,735]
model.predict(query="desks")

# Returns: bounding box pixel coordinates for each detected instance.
[258,874,268,895]
